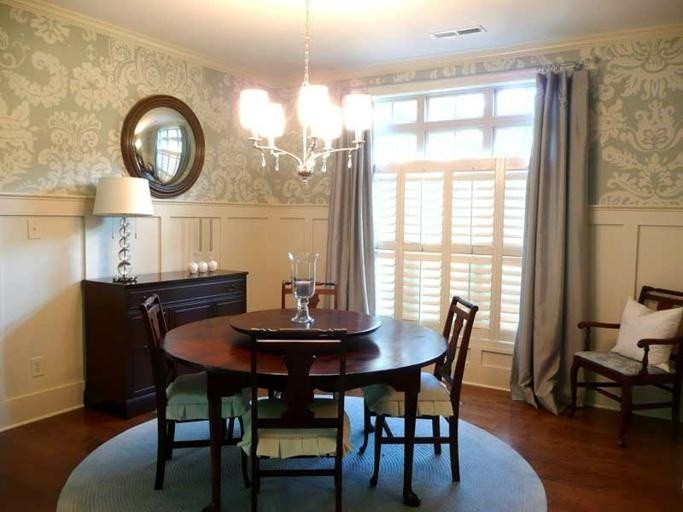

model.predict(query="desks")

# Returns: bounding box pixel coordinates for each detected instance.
[159,306,449,512]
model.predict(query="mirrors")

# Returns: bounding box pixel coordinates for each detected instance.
[120,89,208,198]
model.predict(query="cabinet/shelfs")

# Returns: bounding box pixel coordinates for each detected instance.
[82,266,249,421]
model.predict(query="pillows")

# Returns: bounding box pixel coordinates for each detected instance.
[610,296,682,375]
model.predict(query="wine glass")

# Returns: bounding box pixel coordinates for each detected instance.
[284,250,320,324]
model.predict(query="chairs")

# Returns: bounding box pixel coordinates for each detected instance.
[358,296,480,489]
[563,279,682,449]
[136,288,249,491]
[238,324,353,510]
[266,279,338,403]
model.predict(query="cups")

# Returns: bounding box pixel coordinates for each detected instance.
[188,261,217,275]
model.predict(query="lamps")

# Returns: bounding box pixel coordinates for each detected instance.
[233,2,376,180]
[92,178,157,281]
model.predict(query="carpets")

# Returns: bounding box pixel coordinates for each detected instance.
[53,415,548,512]
